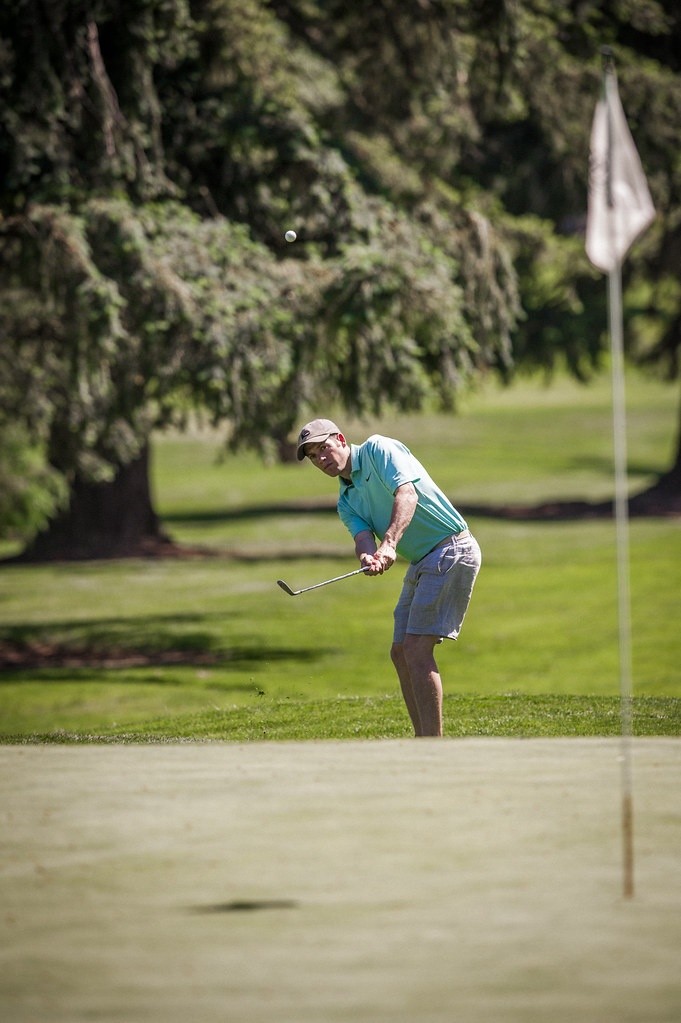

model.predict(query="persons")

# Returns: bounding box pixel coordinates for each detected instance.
[296,419,482,738]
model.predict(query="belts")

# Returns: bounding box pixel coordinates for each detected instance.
[435,530,470,545]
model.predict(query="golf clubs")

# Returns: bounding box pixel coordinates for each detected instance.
[276,565,371,596]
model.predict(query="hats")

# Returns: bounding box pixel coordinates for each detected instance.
[296,418,343,461]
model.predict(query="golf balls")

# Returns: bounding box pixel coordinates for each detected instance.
[285,230,297,242]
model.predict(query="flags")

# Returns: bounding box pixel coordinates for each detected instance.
[585,98,655,271]
